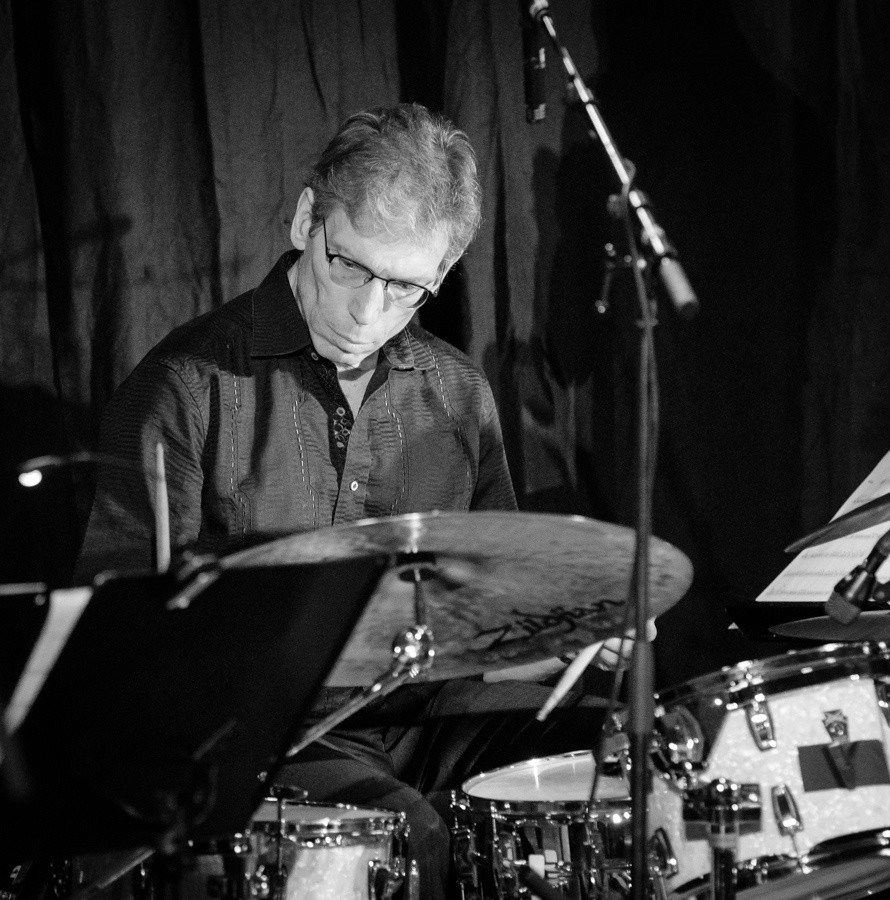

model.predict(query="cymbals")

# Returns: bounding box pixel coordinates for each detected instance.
[786,493,890,554]
[766,611,890,643]
[217,510,692,688]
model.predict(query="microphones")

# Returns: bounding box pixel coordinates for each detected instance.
[520,0,546,123]
[825,531,889,625]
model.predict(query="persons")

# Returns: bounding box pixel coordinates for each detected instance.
[70,104,629,899]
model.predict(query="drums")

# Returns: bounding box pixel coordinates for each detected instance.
[592,638,890,900]
[452,748,634,900]
[140,798,408,900]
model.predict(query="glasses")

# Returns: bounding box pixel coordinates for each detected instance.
[323,217,437,311]
